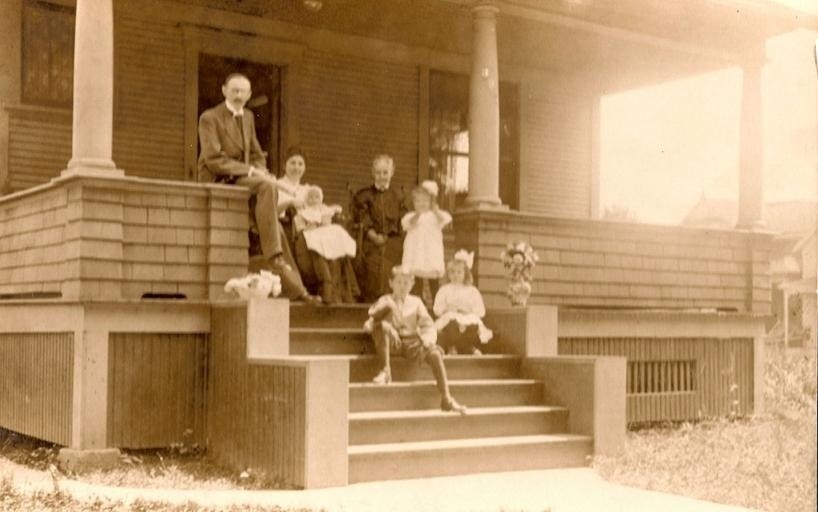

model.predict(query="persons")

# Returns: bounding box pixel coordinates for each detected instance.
[294,180,333,230]
[195,72,325,306]
[276,144,361,304]
[401,180,454,303]
[364,263,467,416]
[433,249,494,356]
[351,153,415,299]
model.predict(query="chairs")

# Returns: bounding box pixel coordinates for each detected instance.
[346,181,406,302]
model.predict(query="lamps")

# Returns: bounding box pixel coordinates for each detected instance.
[304,0,323,13]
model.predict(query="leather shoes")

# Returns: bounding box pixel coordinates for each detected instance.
[268,255,291,273]
[299,290,322,304]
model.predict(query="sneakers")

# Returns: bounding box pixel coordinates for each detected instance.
[371,371,391,385]
[440,398,465,413]
